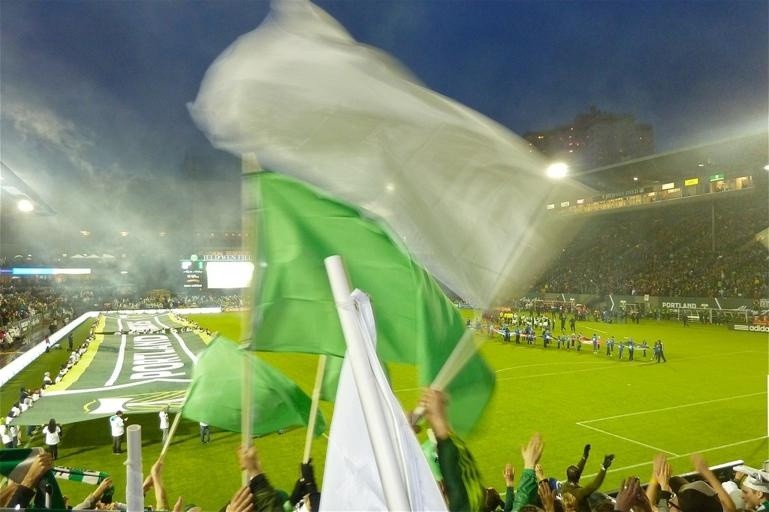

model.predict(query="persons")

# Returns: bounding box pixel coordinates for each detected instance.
[158,405,172,445]
[460,159,768,362]
[0,234,252,362]
[108,411,130,453]
[0,426,769,511]
[0,362,76,460]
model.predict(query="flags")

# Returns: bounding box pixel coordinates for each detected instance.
[171,286,459,511]
[241,170,500,447]
[185,0,590,322]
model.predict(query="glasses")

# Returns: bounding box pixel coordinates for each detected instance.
[666,498,680,511]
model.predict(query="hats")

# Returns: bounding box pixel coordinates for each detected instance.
[667,476,725,511]
[743,470,769,495]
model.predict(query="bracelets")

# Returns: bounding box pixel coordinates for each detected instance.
[601,464,606,471]
[582,456,585,460]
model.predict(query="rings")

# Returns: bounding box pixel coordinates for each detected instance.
[624,486,627,490]
